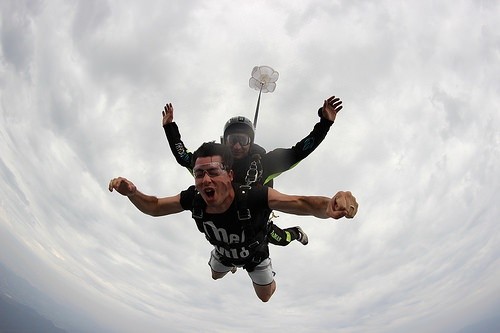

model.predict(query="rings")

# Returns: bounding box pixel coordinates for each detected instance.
[350,204,355,210]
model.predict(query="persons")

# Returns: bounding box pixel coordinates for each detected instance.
[162,96,343,247]
[108,142,359,302]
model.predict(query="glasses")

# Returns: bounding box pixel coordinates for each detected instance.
[193,161,226,178]
[227,134,251,145]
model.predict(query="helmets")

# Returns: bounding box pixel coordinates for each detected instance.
[223,115,255,145]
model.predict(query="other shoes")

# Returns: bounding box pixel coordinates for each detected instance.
[231,265,237,274]
[297,225,308,245]
